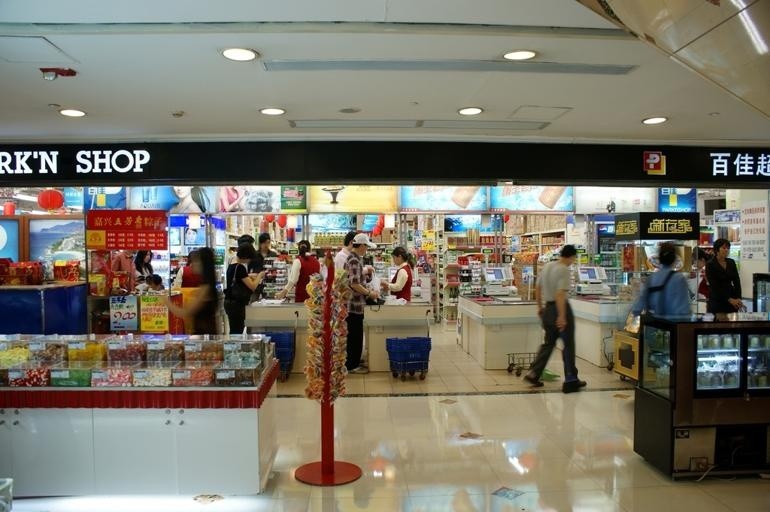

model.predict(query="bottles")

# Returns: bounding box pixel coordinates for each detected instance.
[595,238,633,295]
[697,334,770,388]
[479,235,507,244]
[263,256,289,299]
[448,286,458,320]
[457,256,482,298]
[652,329,672,394]
[718,225,741,275]
[314,232,347,248]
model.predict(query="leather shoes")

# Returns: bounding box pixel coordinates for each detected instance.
[522,374,544,387]
[562,379,587,394]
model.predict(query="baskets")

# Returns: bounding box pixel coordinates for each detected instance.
[246,330,296,374]
[386,333,432,373]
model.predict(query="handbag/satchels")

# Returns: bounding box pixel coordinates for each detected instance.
[696,278,714,301]
[222,263,254,305]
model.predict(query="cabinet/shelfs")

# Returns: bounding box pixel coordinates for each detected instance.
[631,313,770,478]
[308,214,348,260]
[357,214,439,327]
[508,214,567,301]
[438,215,508,322]
[0,332,281,494]
[212,216,301,330]
[568,219,745,304]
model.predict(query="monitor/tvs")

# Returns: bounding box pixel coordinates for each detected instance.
[484,268,505,281]
[577,266,600,282]
[388,267,397,283]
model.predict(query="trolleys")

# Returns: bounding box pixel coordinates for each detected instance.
[383,307,434,383]
[505,351,542,379]
[247,309,301,383]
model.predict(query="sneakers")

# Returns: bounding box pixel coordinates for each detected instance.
[348,366,369,375]
[359,361,369,367]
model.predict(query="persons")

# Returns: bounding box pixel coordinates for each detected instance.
[219,186,248,212]
[111,232,381,375]
[168,185,209,214]
[633,239,740,316]
[524,243,586,393]
[381,247,414,301]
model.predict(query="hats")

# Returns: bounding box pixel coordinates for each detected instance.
[351,233,377,250]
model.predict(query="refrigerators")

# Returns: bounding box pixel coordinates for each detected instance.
[590,213,639,295]
